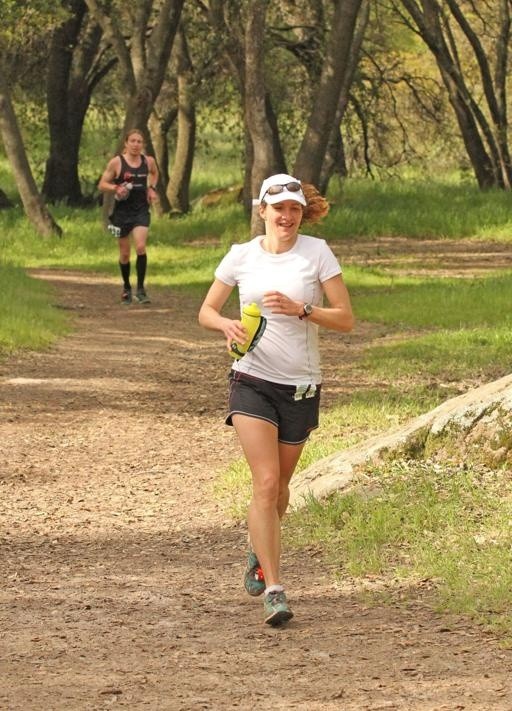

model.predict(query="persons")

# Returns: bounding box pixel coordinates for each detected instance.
[96,129,160,306]
[197,173,355,628]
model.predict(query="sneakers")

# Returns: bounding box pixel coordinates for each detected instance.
[263,589,294,627]
[119,289,151,305]
[244,552,266,597]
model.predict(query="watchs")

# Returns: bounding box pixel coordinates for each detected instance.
[299,302,313,321]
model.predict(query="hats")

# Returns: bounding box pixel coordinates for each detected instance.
[259,174,306,207]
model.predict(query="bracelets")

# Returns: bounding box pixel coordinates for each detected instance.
[149,184,157,192]
[113,185,119,194]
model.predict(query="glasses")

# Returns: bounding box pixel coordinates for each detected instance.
[262,182,302,200]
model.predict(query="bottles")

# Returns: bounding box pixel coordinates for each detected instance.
[113,181,134,203]
[226,301,262,361]
